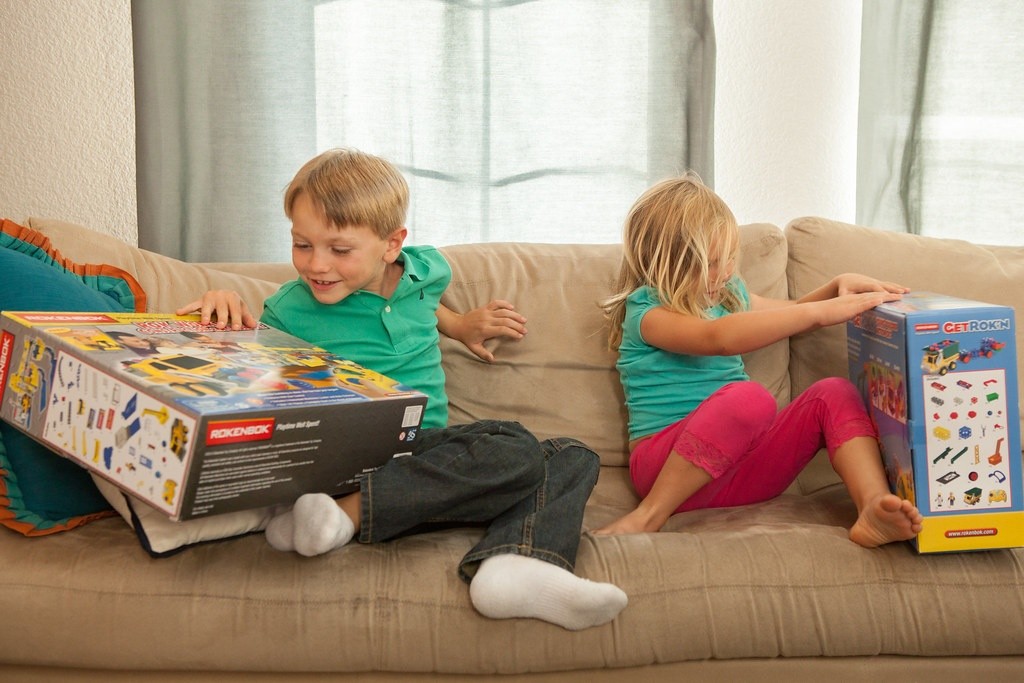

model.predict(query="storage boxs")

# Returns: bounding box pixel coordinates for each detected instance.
[848,293,1023,552]
[0,309,428,520]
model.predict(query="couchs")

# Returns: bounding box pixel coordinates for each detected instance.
[0,206,1023,683]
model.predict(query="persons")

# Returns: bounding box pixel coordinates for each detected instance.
[169,147,627,634]
[581,168,927,548]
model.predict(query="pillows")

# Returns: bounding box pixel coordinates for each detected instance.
[0,218,147,536]
[88,470,295,555]
